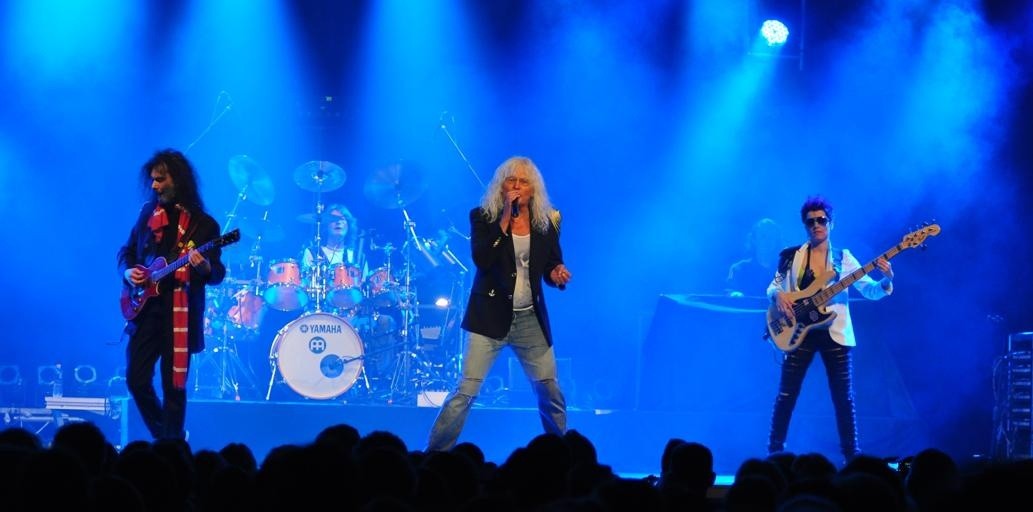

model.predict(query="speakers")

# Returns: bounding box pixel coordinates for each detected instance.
[71,356,112,398]
[0,353,29,408]
[31,356,64,407]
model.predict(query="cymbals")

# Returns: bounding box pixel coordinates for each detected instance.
[229,154,274,205]
[295,160,345,193]
[297,213,341,225]
[364,159,430,208]
[239,218,285,241]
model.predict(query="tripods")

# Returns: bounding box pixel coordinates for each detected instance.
[263,200,374,405]
[356,210,487,407]
[187,193,268,404]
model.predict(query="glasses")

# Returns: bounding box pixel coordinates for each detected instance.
[802,215,829,228]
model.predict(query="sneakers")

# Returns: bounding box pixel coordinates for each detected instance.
[180,430,191,445]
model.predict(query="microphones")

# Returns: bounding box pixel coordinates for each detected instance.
[432,110,451,139]
[224,97,241,123]
[392,157,404,186]
[510,195,521,218]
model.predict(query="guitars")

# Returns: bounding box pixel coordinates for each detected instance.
[120,229,239,322]
[767,219,940,353]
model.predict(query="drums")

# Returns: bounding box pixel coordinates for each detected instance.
[226,289,267,331]
[365,267,397,307]
[264,258,310,312]
[402,236,443,278]
[268,311,366,401]
[326,263,363,308]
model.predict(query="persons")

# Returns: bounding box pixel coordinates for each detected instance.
[725,218,779,298]
[423,156,571,451]
[767,194,895,466]
[116,147,225,442]
[302,203,396,403]
